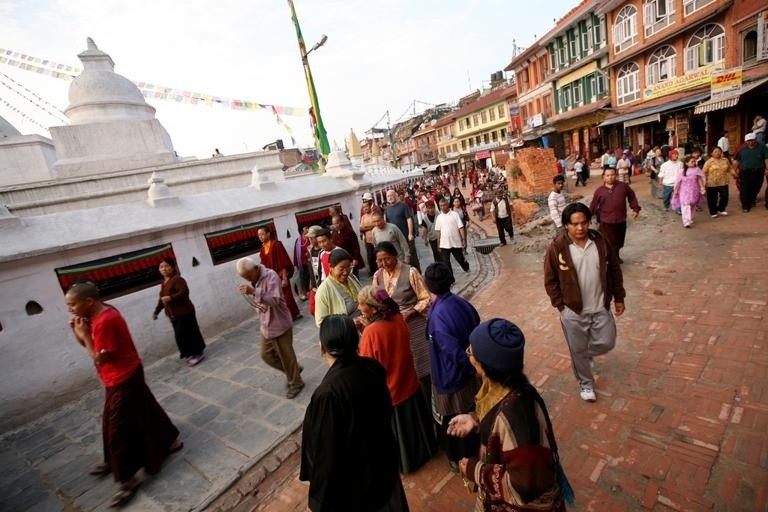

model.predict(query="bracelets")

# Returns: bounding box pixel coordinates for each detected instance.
[282,279,287,281]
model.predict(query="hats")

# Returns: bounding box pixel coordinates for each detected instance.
[361,192,374,200]
[470,317,525,371]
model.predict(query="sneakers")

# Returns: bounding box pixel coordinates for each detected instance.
[292,293,309,321]
[580,385,597,402]
[286,367,305,399]
[185,354,203,367]
[663,206,757,228]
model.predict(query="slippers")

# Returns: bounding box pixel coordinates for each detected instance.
[88,442,184,510]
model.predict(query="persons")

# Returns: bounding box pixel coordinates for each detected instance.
[232,254,305,400]
[421,260,482,479]
[355,283,439,475]
[68,281,122,477]
[63,282,183,509]
[589,167,640,264]
[296,309,410,511]
[548,175,571,239]
[639,112,767,229]
[556,155,590,187]
[601,146,638,185]
[258,136,515,405]
[543,202,626,401]
[444,318,575,512]
[151,255,208,368]
